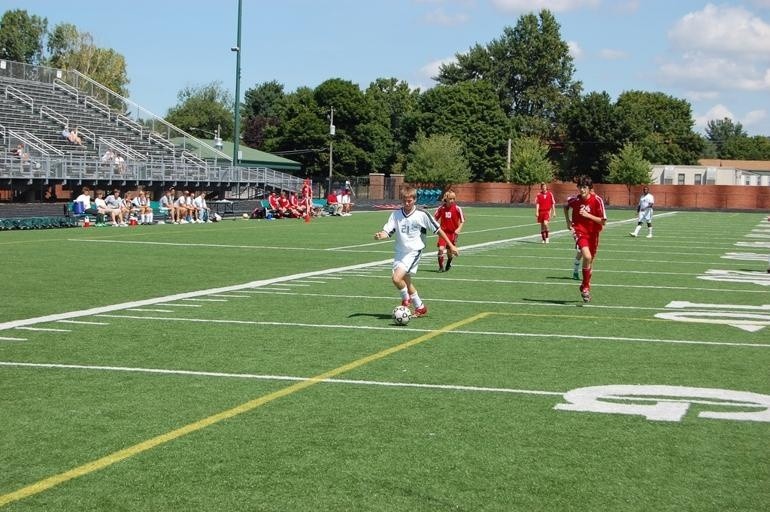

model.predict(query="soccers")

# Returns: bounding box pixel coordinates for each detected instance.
[243,213,248,218]
[392,305,411,325]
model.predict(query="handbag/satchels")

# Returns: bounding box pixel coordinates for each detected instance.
[72,201,84,214]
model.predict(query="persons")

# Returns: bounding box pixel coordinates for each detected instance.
[629,186,654,238]
[432,190,465,273]
[534,182,556,244]
[14,143,29,159]
[374,186,460,318]
[62,125,82,145]
[564,175,607,302]
[102,148,125,172]
[268,181,354,220]
[74,187,213,227]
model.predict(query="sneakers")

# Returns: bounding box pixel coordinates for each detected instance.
[333,212,352,216]
[629,232,652,238]
[172,219,213,224]
[279,213,303,219]
[94,222,155,227]
[541,237,549,243]
[573,272,592,303]
[438,261,452,273]
[402,297,427,318]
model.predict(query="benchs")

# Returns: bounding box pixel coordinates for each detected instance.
[66,200,169,226]
[259,199,328,218]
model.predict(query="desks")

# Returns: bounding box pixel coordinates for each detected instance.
[206,200,238,222]
